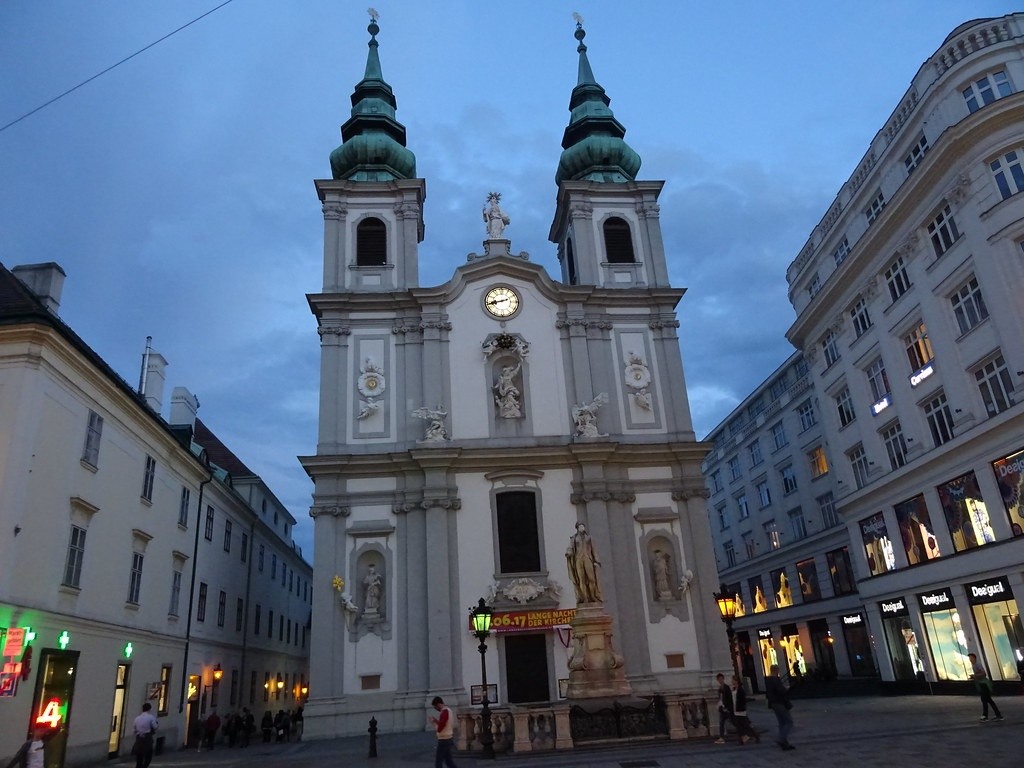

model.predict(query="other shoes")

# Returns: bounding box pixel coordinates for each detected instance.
[714,738,726,744]
[275,741,280,745]
[754,737,760,744]
[774,739,788,749]
[267,742,272,745]
[782,744,796,751]
[736,739,743,746]
[263,742,268,745]
[742,734,750,743]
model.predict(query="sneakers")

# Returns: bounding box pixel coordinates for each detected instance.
[991,716,1005,722]
[980,717,990,723]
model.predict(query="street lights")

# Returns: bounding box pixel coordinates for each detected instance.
[712,582,741,687]
[470,597,496,760]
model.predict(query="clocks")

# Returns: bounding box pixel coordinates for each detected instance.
[480,282,523,322]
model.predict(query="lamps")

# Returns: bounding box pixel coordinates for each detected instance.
[272,678,284,697]
[297,683,308,702]
[203,664,223,693]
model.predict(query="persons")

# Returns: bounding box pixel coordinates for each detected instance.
[6,722,62,768]
[952,613,971,676]
[483,339,527,399]
[971,501,990,544]
[564,522,604,602]
[969,654,1004,721]
[576,402,595,425]
[715,674,762,745]
[882,536,896,571]
[133,703,157,768]
[766,665,797,750]
[481,192,511,238]
[192,706,303,753]
[654,549,673,597]
[428,697,457,768]
[901,620,927,679]
[679,572,692,593]
[425,403,448,434]
[361,566,383,609]
